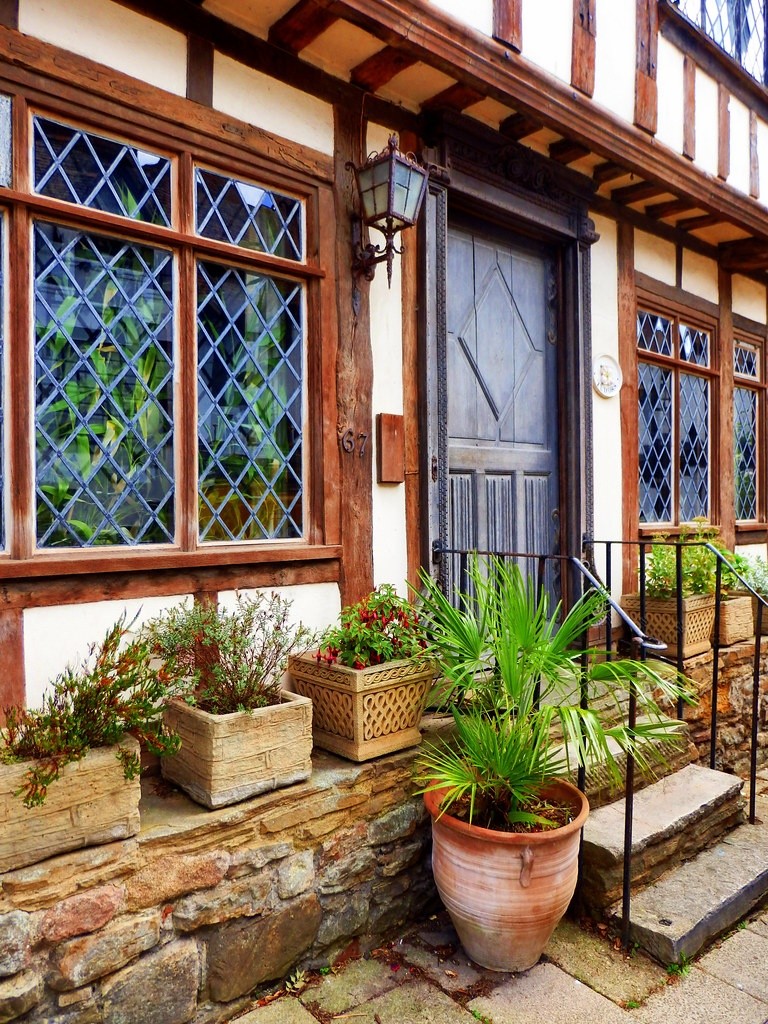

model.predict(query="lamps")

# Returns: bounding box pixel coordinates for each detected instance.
[343,133,438,290]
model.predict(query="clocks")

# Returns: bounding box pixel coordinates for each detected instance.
[593,354,624,399]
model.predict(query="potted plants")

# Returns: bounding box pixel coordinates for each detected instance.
[0,583,434,873]
[388,548,701,974]
[620,516,768,659]
